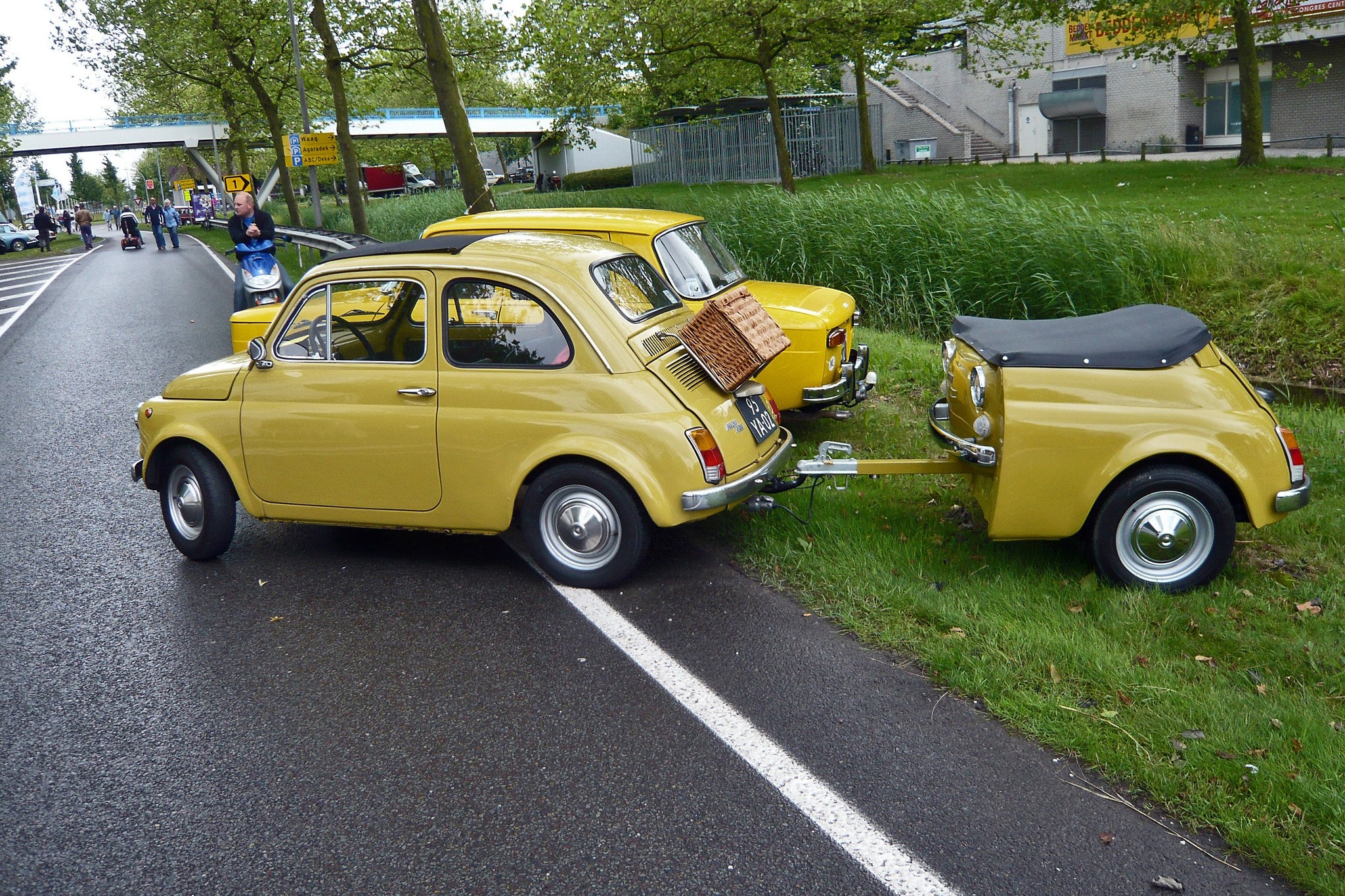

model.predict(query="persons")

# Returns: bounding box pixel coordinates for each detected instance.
[63,210,72,236]
[144,198,166,250]
[120,205,145,245]
[162,199,182,248]
[73,205,96,241]
[70,212,80,232]
[103,208,112,231]
[75,203,93,250]
[58,215,63,226]
[227,191,294,313]
[111,206,121,230]
[34,206,54,252]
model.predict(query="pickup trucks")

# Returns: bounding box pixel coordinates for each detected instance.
[483,168,512,186]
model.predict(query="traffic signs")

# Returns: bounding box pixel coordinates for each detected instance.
[281,133,336,147]
[285,153,339,167]
[224,174,252,194]
[283,146,338,157]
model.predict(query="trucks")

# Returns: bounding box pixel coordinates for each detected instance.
[365,161,436,200]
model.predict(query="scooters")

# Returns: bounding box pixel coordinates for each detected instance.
[224,234,294,309]
[533,170,561,193]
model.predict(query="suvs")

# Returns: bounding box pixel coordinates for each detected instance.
[509,166,535,184]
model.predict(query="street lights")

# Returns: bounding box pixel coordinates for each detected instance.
[123,168,150,207]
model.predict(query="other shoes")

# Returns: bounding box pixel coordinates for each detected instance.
[141,242,144,245]
[162,246,166,249]
[86,247,89,249]
[88,242,93,248]
[173,246,179,248]
[159,247,161,249]
[41,246,44,252]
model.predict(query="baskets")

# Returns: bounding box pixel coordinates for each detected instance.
[677,286,792,392]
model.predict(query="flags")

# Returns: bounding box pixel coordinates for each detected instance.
[13,166,36,215]
[51,185,67,201]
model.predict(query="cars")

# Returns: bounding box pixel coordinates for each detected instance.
[0,225,39,254]
[227,207,878,422]
[160,205,208,226]
[18,215,62,235]
[129,235,796,589]
[141,207,147,216]
[9,223,57,248]
[55,209,76,221]
